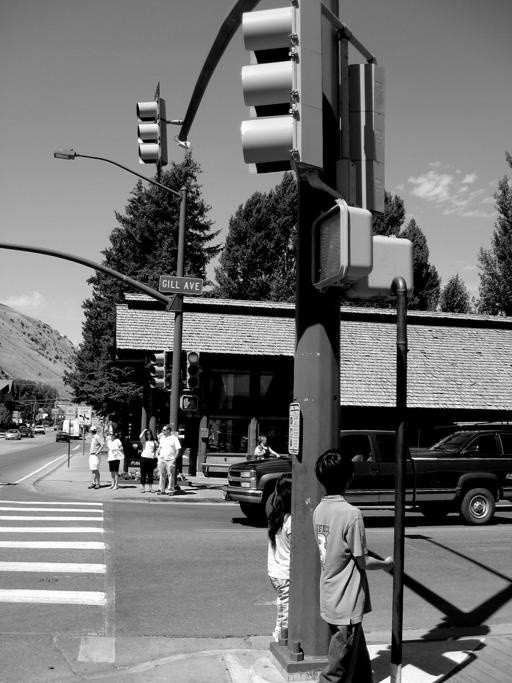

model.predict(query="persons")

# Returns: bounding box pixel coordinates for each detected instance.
[311,448,374,683]
[87,423,182,496]
[253,436,281,461]
[266,471,292,642]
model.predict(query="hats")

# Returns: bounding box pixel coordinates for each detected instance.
[90,425,97,432]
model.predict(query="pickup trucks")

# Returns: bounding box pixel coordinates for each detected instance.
[223,430,511,530]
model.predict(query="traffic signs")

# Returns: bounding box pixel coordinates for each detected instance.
[158,271,205,295]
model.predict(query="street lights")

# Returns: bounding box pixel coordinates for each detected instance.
[54,151,190,482]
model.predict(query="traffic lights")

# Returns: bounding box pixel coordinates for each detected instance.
[311,205,367,281]
[136,98,161,160]
[242,6,297,161]
[178,393,199,411]
[184,349,201,389]
[150,348,172,393]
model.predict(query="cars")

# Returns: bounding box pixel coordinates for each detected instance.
[35,425,44,434]
[19,426,35,437]
[4,429,20,441]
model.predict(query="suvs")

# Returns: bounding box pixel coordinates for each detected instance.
[427,419,512,520]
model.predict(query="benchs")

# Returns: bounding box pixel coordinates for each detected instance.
[200,461,231,476]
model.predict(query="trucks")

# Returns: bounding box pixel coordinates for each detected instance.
[52,406,97,441]
